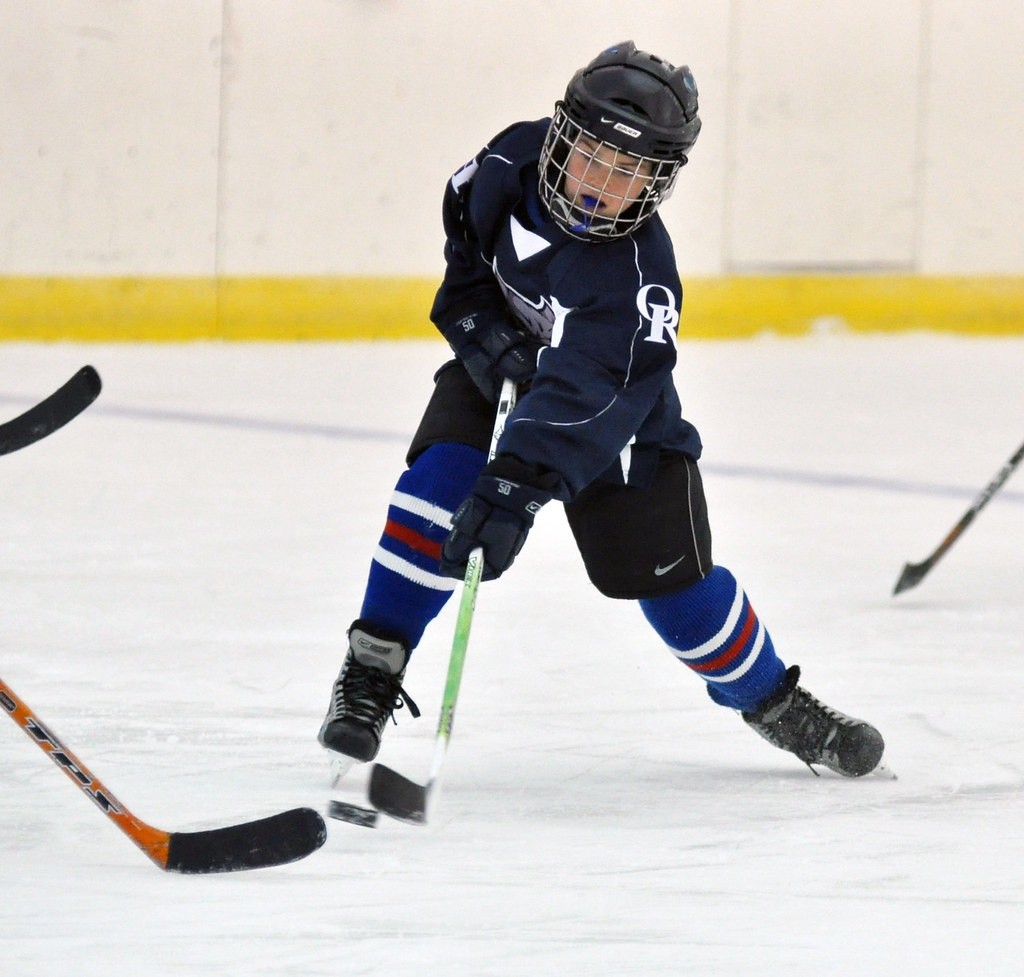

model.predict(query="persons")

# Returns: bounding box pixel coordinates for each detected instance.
[318,39,885,779]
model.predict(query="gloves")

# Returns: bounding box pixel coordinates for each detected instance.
[444,309,536,405]
[438,455,562,582]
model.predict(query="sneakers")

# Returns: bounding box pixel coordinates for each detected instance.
[742,664,899,779]
[316,617,422,788]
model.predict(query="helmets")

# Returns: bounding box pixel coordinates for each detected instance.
[537,39,702,243]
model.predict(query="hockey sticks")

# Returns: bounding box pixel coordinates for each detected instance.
[892,442,1023,597]
[0,678,327,875]
[367,373,517,828]
[0,364,102,458]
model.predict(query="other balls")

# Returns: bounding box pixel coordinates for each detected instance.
[328,798,378,829]
